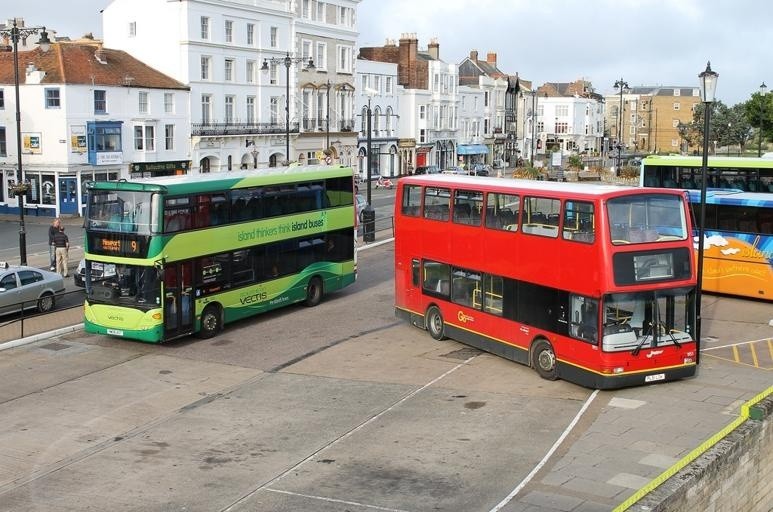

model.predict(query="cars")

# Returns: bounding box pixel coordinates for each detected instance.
[413,162,490,178]
[0,260,65,317]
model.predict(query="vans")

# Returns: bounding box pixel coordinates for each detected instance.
[72,256,117,291]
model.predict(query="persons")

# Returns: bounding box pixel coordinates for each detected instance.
[577,302,604,343]
[101,267,127,288]
[327,239,336,259]
[266,264,279,277]
[379,175,383,191]
[52,226,70,278]
[47,219,60,272]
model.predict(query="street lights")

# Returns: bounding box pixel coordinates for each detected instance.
[258,51,316,169]
[0,19,51,267]
[518,88,548,169]
[757,81,767,157]
[315,78,346,158]
[613,78,631,175]
[694,60,719,364]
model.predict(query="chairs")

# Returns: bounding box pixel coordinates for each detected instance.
[104,194,282,232]
[423,203,659,245]
[435,277,467,306]
[645,176,772,194]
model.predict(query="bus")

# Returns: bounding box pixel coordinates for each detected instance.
[80,163,359,345]
[638,154,772,306]
[393,171,700,391]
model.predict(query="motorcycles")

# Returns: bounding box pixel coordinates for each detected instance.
[374,176,393,189]
